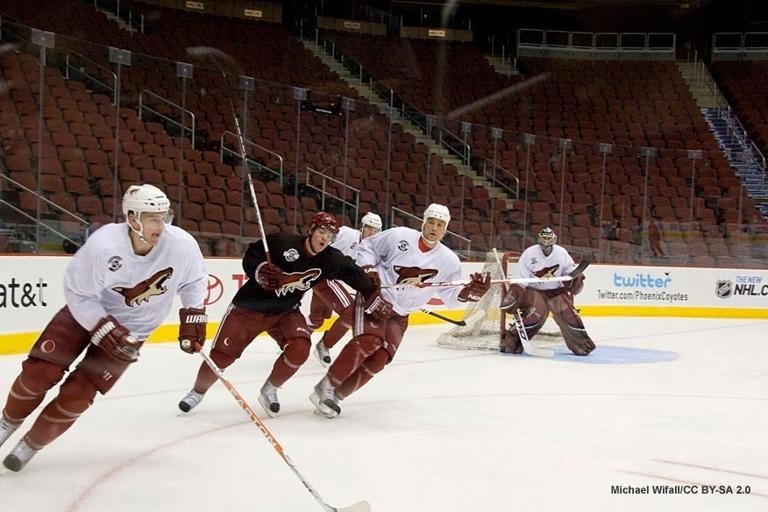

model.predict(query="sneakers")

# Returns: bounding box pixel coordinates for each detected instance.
[0,417,22,450]
[314,376,336,413]
[334,405,341,417]
[179,389,204,413]
[2,439,38,474]
[260,377,280,414]
[316,339,331,363]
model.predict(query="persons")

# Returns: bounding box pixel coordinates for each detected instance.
[0,184,203,472]
[302,211,382,368]
[502,227,593,358]
[178,210,375,417]
[309,203,491,420]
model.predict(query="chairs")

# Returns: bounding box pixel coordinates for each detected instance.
[498,58,768,268]
[0,0,499,251]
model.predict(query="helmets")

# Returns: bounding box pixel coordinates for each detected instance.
[307,210,338,254]
[359,211,382,242]
[122,183,172,248]
[536,227,556,248]
[421,202,449,244]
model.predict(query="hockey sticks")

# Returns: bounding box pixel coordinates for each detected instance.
[193,342,371,512]
[207,47,273,267]
[377,255,594,289]
[420,306,486,328]
[492,247,553,357]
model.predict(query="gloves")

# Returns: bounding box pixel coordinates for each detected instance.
[458,272,491,304]
[361,291,393,322]
[255,262,282,294]
[88,316,139,364]
[360,265,381,290]
[177,308,206,355]
